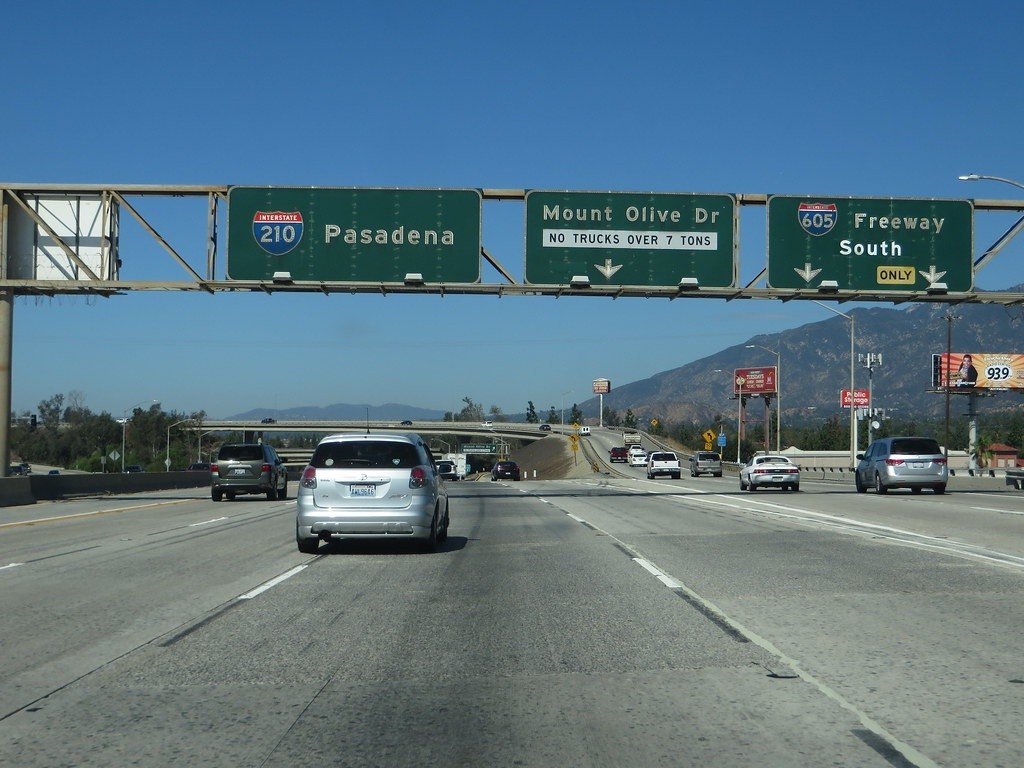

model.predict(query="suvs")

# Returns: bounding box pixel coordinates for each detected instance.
[435,460,457,481]
[689,452,723,478]
[855,436,948,495]
[211,442,288,502]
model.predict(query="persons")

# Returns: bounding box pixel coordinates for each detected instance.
[955,355,978,387]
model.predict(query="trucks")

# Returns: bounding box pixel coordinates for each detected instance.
[442,453,466,480]
[622,428,641,450]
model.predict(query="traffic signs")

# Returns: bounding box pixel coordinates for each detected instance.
[523,189,737,289]
[766,194,974,293]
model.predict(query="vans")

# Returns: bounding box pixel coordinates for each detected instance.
[579,427,590,436]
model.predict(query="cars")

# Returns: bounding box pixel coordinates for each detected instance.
[539,425,551,431]
[116,418,130,424]
[481,421,493,428]
[19,463,31,473]
[400,420,412,425]
[188,462,211,471]
[739,456,800,492]
[624,445,660,467]
[296,432,450,553]
[124,465,145,474]
[491,461,520,481]
[48,470,61,476]
[9,465,27,476]
[261,417,277,424]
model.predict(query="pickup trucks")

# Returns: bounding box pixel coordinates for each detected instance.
[608,447,627,463]
[644,452,681,480]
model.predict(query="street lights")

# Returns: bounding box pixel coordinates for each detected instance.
[430,438,450,453]
[857,353,883,448]
[166,418,196,471]
[939,315,962,467]
[713,370,742,464]
[744,343,780,456]
[121,399,158,473]
